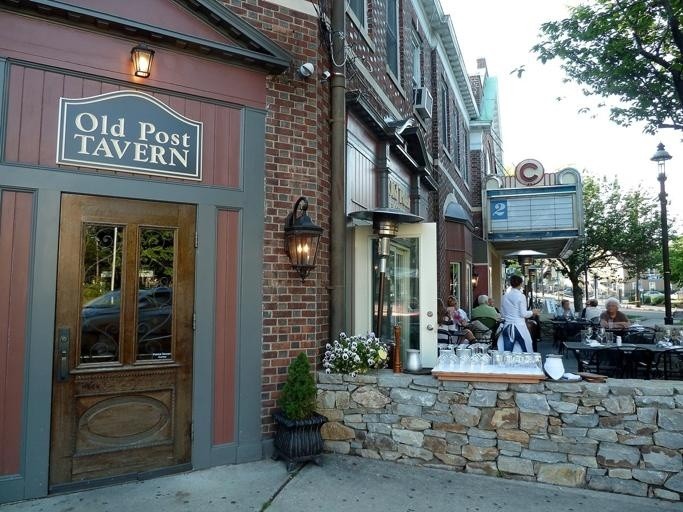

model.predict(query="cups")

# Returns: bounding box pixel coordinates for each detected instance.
[605,332,613,346]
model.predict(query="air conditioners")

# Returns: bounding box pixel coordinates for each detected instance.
[413,86,433,119]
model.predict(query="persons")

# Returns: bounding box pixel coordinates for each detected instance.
[599,297,631,329]
[554,300,574,347]
[584,299,602,322]
[441,295,501,349]
[501,275,540,352]
[581,300,590,320]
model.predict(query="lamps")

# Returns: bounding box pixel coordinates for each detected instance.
[129,42,156,78]
[472,264,480,288]
[283,196,324,283]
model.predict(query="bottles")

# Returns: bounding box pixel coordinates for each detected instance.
[404,348,422,372]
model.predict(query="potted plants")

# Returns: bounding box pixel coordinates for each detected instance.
[269,351,329,471]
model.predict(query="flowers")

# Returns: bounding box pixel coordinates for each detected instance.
[322,331,390,378]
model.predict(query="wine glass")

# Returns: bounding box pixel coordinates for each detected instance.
[436,342,542,372]
[597,327,606,345]
[584,326,593,345]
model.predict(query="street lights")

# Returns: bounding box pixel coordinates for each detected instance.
[651,143,673,324]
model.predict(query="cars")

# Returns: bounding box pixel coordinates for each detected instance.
[670,291,683,300]
[641,290,664,303]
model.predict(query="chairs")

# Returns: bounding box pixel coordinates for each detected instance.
[437,315,683,381]
